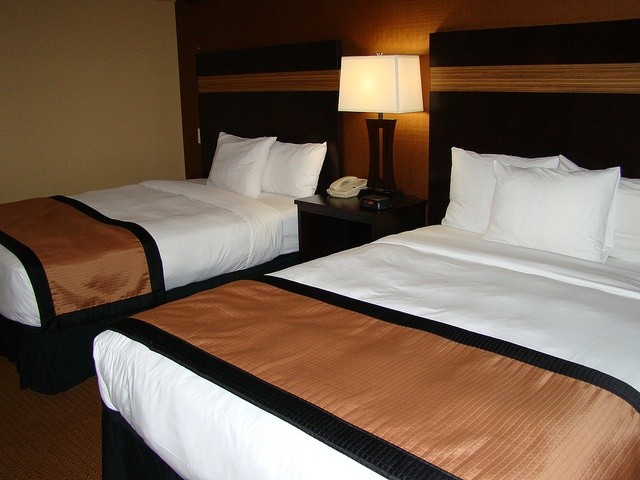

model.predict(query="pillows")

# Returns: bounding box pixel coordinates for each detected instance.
[608,177,639,266]
[205,131,278,200]
[439,147,558,234]
[261,139,327,199]
[482,158,621,264]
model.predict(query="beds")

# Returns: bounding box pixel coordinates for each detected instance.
[0,93,343,395]
[92,88,640,479]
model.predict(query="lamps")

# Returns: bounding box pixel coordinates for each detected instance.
[337,53,424,203]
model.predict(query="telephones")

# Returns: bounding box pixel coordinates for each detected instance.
[327,176,368,199]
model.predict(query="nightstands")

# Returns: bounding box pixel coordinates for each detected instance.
[294,189,427,261]
